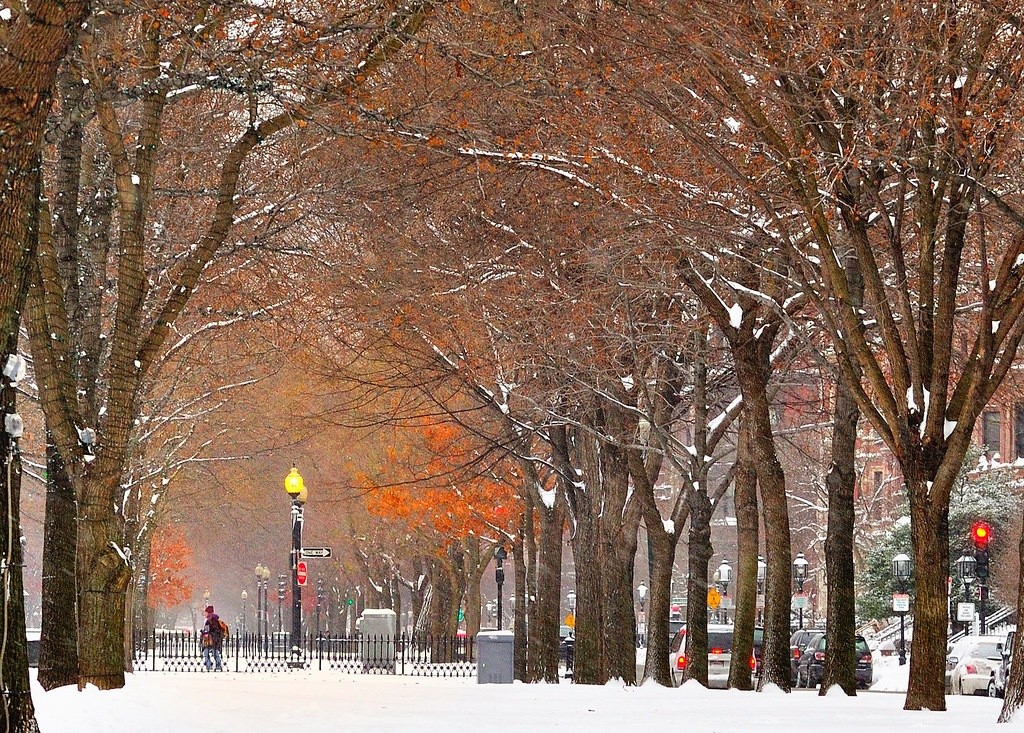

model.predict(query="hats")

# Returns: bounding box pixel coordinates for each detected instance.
[205,606,213,613]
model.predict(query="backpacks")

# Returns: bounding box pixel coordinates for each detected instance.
[211,618,228,638]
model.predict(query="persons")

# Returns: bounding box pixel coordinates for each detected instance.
[200,606,228,670]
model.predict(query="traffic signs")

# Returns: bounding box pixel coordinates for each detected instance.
[300,546,332,558]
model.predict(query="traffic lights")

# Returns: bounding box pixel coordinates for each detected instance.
[974,523,990,579]
[495,547,508,559]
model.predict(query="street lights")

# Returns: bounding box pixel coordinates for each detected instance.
[205,590,210,611]
[567,589,577,631]
[263,566,270,651]
[718,559,731,622]
[241,590,247,651]
[957,548,976,633]
[893,552,910,666]
[638,582,647,648]
[255,563,264,652]
[485,596,515,629]
[794,552,807,630]
[284,464,309,668]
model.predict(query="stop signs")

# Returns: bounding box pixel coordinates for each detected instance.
[294,562,308,589]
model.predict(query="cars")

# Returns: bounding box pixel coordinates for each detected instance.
[668,625,755,691]
[755,630,873,692]
[946,630,1016,700]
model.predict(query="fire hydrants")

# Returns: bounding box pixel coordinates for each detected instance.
[563,633,575,677]
